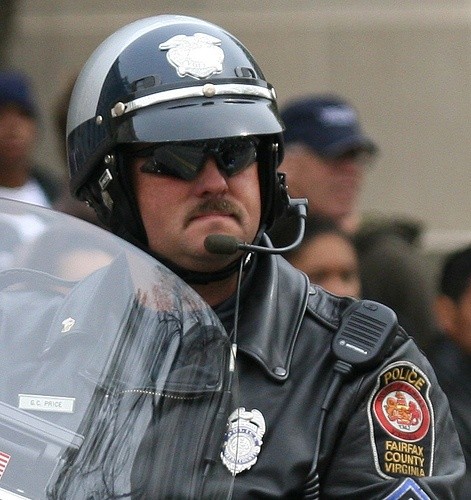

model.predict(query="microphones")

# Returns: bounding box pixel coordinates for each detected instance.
[204,198,308,255]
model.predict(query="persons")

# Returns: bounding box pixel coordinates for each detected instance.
[272,93,471,469]
[0,65,66,208]
[0,14,471,500]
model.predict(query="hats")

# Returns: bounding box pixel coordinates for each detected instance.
[280,96,377,162]
[1,72,38,116]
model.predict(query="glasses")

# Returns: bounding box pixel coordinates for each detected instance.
[132,136,268,177]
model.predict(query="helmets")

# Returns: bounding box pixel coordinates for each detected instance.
[64,15,289,199]
